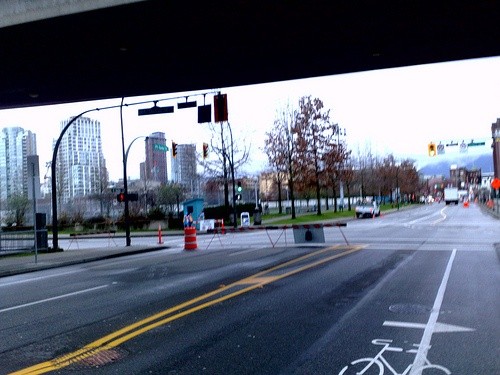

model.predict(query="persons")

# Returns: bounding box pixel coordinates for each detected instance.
[259,204,263,215]
[183,212,193,228]
[338,199,344,213]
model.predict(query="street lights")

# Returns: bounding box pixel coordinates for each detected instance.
[253,175,258,210]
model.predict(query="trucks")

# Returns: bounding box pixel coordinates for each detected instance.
[444,187,459,205]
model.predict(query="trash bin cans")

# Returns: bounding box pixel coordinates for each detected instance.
[36,229,48,249]
[254,209,261,224]
[240,212,250,227]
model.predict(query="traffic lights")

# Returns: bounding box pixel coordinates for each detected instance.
[172,143,178,156]
[237,181,242,191]
[117,193,139,201]
[429,145,437,156]
[203,143,208,159]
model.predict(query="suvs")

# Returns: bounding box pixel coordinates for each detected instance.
[355,200,380,219]
